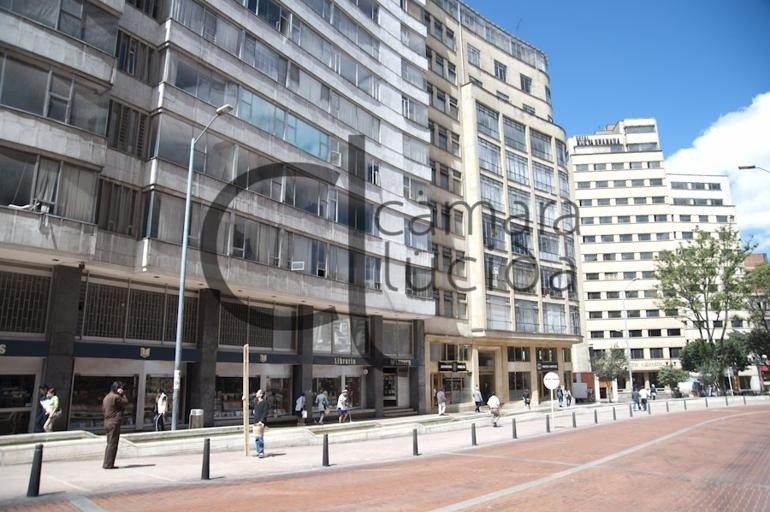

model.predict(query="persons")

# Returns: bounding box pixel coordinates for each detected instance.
[565,387,572,407]
[557,386,563,407]
[522,388,530,409]
[295,389,353,426]
[154,388,168,431]
[434,386,447,416]
[242,389,268,458]
[632,384,656,411]
[36,384,60,432]
[588,387,592,402]
[486,391,501,427]
[472,387,484,412]
[101,381,129,469]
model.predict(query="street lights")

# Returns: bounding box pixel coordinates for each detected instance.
[738,165,770,174]
[171,104,234,431]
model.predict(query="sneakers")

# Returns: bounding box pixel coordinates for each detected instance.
[256,452,264,458]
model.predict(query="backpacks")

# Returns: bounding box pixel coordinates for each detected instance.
[557,390,570,398]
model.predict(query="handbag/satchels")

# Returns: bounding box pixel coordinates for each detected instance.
[526,399,530,403]
[324,408,330,416]
[302,410,308,418]
[50,409,63,422]
